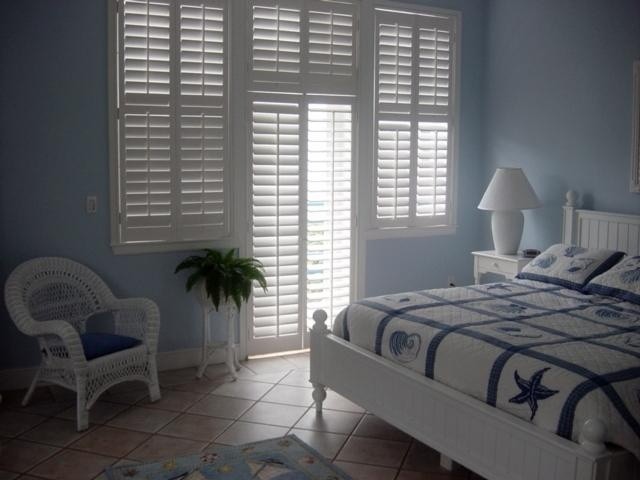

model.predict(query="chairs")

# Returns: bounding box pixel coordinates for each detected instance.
[4,256,161,433]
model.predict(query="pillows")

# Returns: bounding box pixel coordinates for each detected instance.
[515,243,627,293]
[583,255,640,305]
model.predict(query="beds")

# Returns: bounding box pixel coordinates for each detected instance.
[308,189,640,480]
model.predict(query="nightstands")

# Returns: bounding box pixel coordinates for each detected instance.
[470,249,542,283]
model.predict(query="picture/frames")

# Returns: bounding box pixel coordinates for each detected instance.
[632,62,640,192]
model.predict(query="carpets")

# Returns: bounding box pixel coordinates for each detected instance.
[104,434,354,479]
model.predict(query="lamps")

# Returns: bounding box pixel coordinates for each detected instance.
[475,166,542,253]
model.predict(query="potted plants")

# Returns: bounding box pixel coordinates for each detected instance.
[173,248,269,312]
